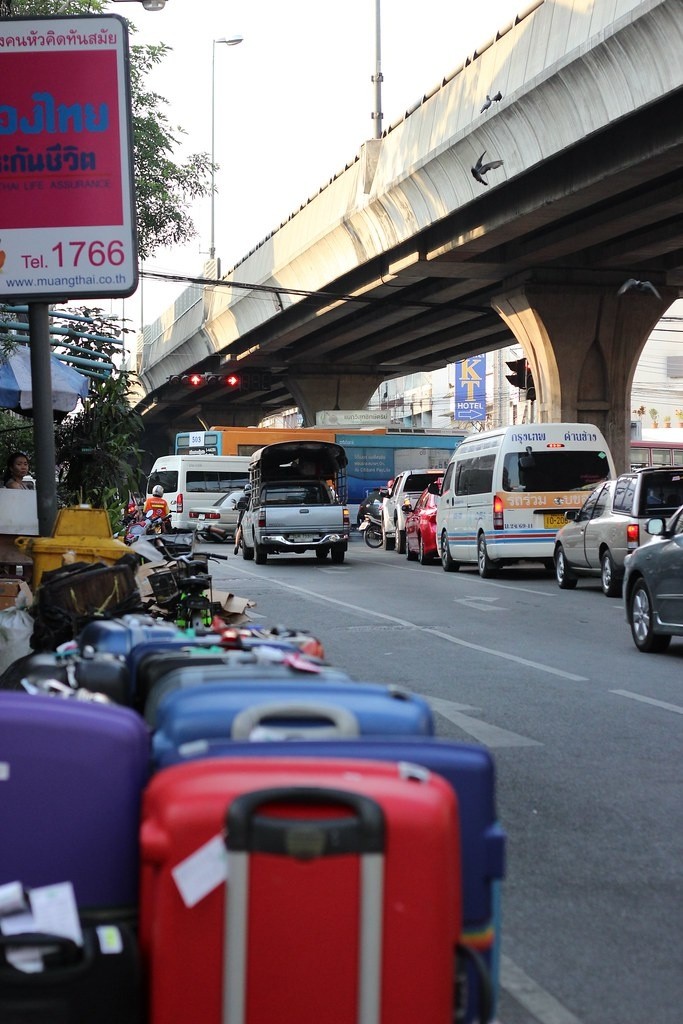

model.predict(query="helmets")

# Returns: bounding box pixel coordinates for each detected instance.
[244,484,251,490]
[152,485,164,497]
[387,479,395,488]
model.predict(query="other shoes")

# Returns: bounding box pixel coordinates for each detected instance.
[233,545,239,555]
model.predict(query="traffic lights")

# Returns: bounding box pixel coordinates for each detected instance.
[504,358,526,389]
[169,375,238,386]
[525,360,536,401]
[241,368,272,390]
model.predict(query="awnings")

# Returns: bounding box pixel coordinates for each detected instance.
[0,345,89,426]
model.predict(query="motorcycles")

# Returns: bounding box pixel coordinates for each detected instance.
[231,498,248,549]
[358,504,383,549]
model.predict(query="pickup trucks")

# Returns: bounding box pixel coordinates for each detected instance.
[552,464,683,598]
[235,440,355,565]
[378,468,446,554]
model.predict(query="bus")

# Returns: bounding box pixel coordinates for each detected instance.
[174,426,470,529]
[630,440,683,472]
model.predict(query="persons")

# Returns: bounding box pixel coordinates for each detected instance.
[143,485,170,532]
[3,452,29,490]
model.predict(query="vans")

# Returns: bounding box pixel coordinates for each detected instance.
[428,422,617,581]
[146,454,251,541]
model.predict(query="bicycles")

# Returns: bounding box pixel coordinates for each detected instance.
[138,523,229,633]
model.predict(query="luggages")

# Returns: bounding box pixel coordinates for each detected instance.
[0,605,506,1024]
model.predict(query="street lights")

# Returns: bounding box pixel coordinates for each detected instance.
[209,33,245,260]
[53,0,169,15]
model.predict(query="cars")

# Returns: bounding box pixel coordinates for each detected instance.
[623,503,683,653]
[116,489,146,516]
[21,476,65,490]
[187,491,247,534]
[402,477,444,565]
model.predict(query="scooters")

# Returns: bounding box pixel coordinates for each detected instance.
[114,509,173,567]
